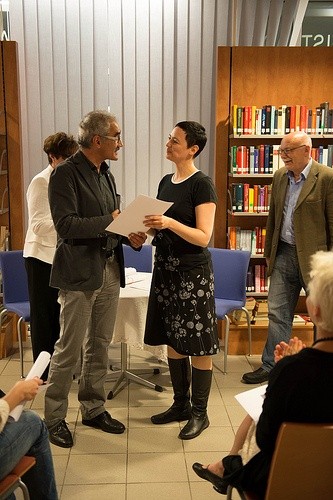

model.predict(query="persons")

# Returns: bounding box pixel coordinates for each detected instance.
[192,249,333,500]
[23,132,79,388]
[143,121,218,440]
[48,111,147,448]
[0,378,57,500]
[242,131,333,384]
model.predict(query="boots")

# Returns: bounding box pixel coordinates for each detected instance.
[179,366,214,439]
[150,356,193,424]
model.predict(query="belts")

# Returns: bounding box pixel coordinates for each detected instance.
[106,249,117,259]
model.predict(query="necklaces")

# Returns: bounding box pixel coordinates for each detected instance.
[310,336,333,347]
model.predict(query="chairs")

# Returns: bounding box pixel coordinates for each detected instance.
[242,424,333,500]
[122,245,153,273]
[0,247,30,378]
[209,247,254,376]
[1,456,37,500]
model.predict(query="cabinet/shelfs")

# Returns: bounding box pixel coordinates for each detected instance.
[215,44,333,354]
[0,40,29,358]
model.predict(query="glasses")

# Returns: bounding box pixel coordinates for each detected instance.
[103,136,119,144]
[278,145,305,154]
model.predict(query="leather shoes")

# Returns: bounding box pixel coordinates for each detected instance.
[192,462,227,494]
[50,421,73,447]
[82,412,124,433]
[243,367,268,383]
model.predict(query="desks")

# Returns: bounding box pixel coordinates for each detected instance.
[105,271,167,400]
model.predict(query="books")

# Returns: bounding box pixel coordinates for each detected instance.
[249,144,285,174]
[291,102,333,134]
[227,297,255,325]
[250,299,270,326]
[232,182,254,212]
[262,105,290,135]
[227,225,267,254]
[230,146,250,174]
[245,264,271,293]
[254,184,273,213]
[291,315,315,327]
[309,144,333,167]
[231,102,262,135]
